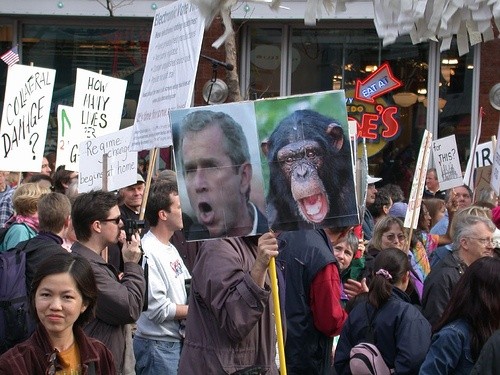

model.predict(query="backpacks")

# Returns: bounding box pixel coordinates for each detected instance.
[349,301,396,375]
[0,240,54,354]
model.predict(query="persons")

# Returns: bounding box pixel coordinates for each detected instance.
[71,190,145,375]
[0,253,119,375]
[276,226,370,375]
[133,182,192,375]
[108,162,205,280]
[180,111,269,240]
[179,233,287,375]
[334,168,500,375]
[0,153,78,355]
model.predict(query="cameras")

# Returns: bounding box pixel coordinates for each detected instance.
[126,218,146,243]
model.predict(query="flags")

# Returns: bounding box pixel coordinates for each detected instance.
[1,46,19,66]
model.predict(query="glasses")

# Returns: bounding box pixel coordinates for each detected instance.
[470,238,495,246]
[101,215,122,225]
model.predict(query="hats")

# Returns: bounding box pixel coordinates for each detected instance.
[367,174,383,185]
[136,173,146,183]
[388,202,407,218]
